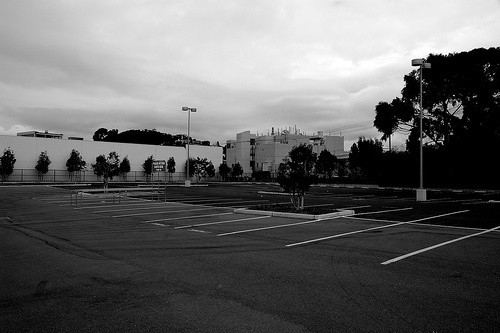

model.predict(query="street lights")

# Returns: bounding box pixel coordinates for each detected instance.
[412,58,431,202]
[182,106,197,188]
